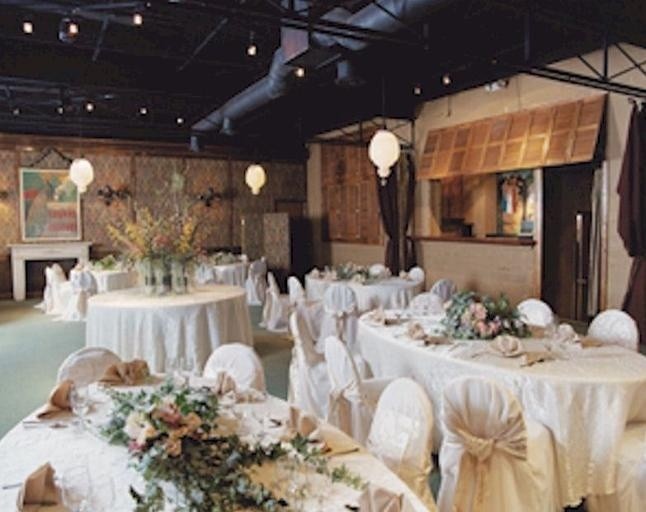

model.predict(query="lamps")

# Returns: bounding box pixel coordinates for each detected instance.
[68,111,94,192]
[367,75,401,190]
[244,151,268,196]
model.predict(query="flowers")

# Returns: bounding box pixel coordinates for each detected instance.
[101,159,225,266]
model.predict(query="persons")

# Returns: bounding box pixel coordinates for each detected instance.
[499,173,529,235]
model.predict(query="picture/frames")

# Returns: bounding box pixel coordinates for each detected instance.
[15,165,84,242]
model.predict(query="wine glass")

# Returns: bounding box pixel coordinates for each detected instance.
[390,289,406,330]
[59,352,334,509]
